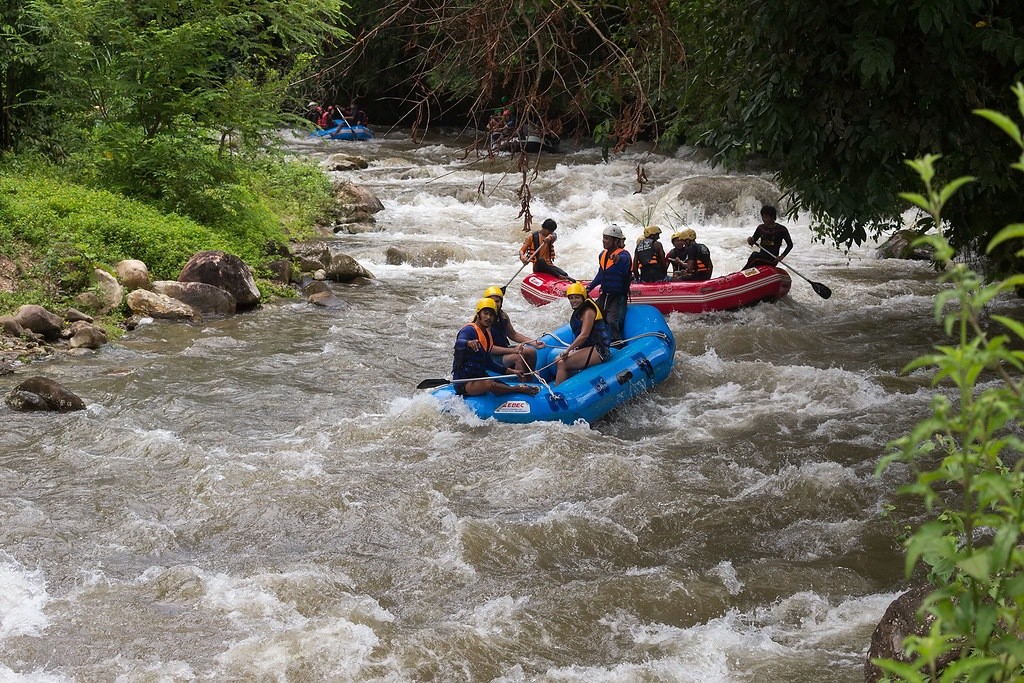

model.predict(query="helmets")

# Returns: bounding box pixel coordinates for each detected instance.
[476,298,497,315]
[635,237,646,246]
[678,229,696,240]
[602,225,622,239]
[567,282,587,299]
[484,287,503,301]
[671,233,680,244]
[644,225,662,238]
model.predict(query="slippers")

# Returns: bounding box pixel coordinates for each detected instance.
[520,384,540,395]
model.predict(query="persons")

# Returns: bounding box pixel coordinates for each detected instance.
[554,283,611,385]
[632,224,713,284]
[519,218,576,284]
[308,101,367,138]
[471,283,546,383]
[586,224,632,350]
[738,204,793,271]
[489,110,518,138]
[453,297,540,397]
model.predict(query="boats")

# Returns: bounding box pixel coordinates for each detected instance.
[521,265,792,316]
[310,125,373,141]
[431,304,676,426]
[500,135,555,153]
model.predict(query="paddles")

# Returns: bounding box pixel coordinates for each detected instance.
[337,107,358,140]
[753,241,832,300]
[416,372,523,389]
[500,240,546,295]
